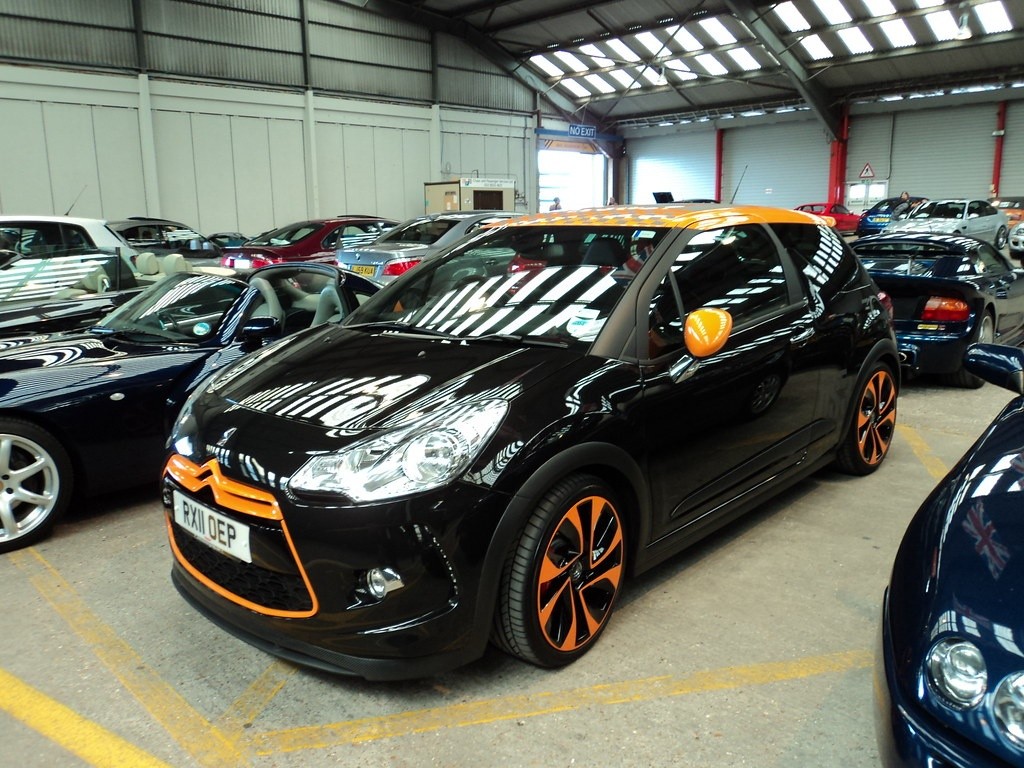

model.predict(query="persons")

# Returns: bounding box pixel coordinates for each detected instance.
[551,197,561,211]
[609,197,619,205]
[899,191,928,213]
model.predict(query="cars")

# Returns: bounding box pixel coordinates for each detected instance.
[161,202,908,682]
[0,215,141,274]
[334,210,530,287]
[856,196,929,237]
[224,215,400,269]
[879,199,1008,250]
[108,215,226,265]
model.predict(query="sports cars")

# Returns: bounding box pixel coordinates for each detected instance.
[794,203,860,234]
[1,268,386,553]
[847,232,1024,389]
[987,197,1024,230]
[0,251,239,313]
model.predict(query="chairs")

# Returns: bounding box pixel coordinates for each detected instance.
[501,238,563,312]
[310,287,344,329]
[244,278,284,332]
[545,236,630,317]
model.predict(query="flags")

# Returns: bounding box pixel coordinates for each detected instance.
[961,499,1010,580]
